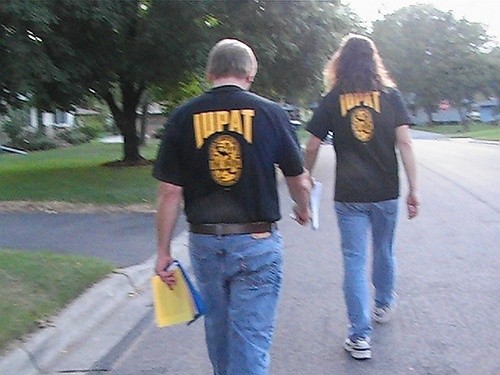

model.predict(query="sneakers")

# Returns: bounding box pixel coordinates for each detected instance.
[372,290,398,323]
[343,338,372,360]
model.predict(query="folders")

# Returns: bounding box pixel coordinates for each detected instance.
[164,258,207,329]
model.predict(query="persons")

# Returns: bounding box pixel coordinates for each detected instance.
[149,39,309,375]
[303,35,420,360]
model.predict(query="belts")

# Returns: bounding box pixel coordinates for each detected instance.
[190,222,277,235]
